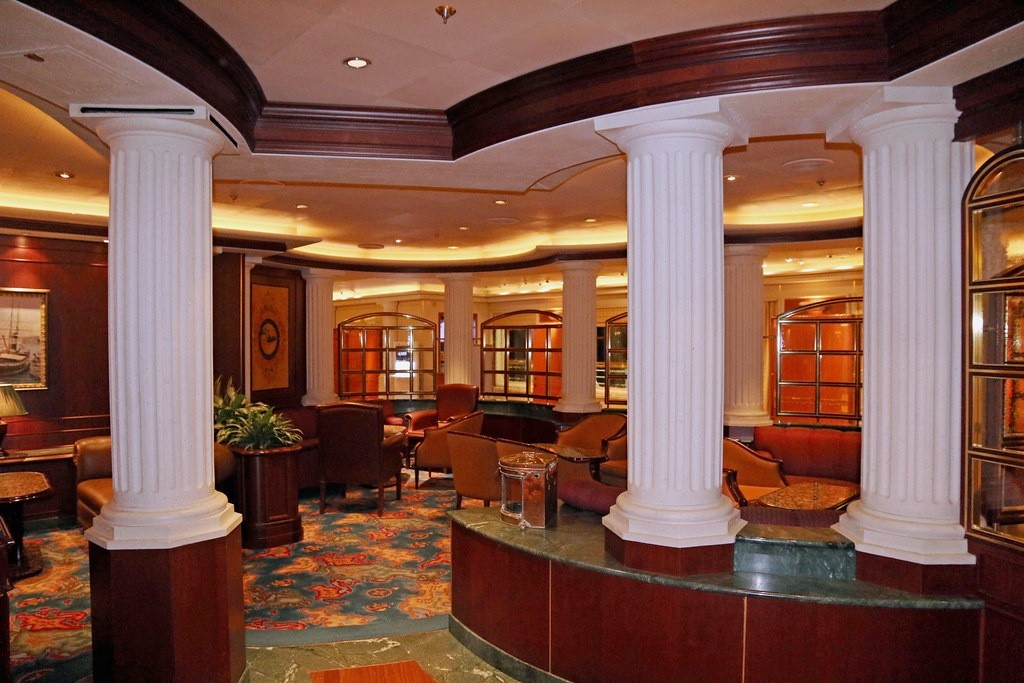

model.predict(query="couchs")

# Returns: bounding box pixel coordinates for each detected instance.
[73,435,232,535]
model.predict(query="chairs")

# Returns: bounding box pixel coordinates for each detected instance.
[274,381,863,518]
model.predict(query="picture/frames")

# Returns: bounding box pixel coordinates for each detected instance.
[0,287,51,391]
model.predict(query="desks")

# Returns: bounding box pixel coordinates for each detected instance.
[0,515,15,683]
[759,481,861,509]
[231,443,304,550]
[0,472,56,581]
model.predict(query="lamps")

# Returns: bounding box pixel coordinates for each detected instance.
[0,383,28,457]
